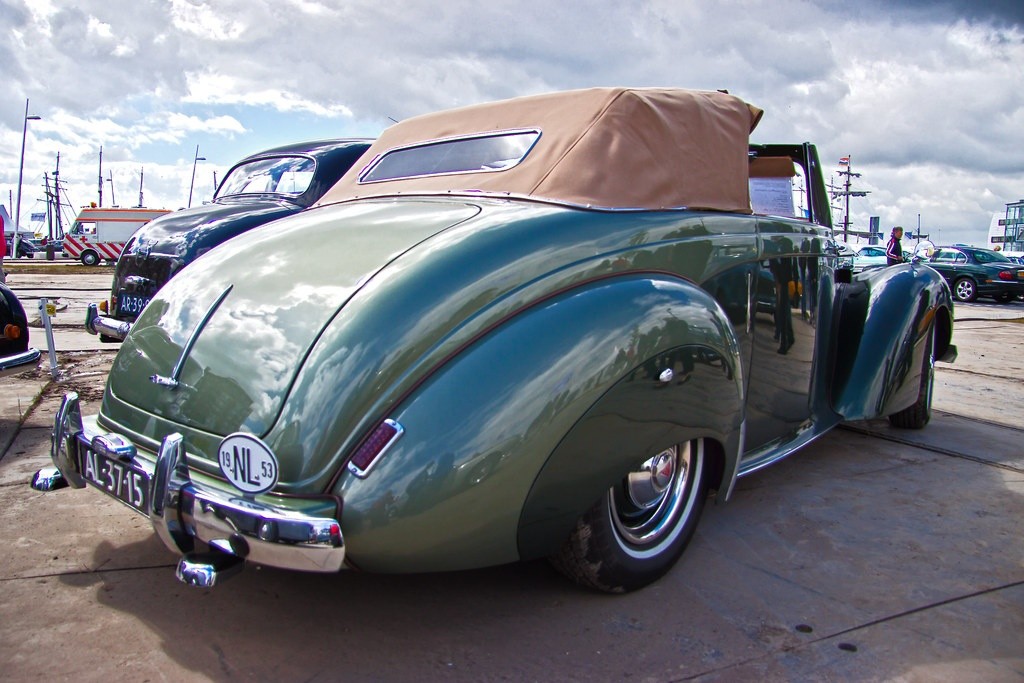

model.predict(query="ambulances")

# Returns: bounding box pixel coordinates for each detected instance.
[60,203,176,267]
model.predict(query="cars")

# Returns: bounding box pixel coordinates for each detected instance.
[850,244,915,274]
[922,245,1023,305]
[77,135,385,341]
[28,82,959,597]
[48,240,64,251]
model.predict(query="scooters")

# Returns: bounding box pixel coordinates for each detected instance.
[10,232,37,259]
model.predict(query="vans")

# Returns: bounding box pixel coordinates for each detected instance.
[27,239,48,251]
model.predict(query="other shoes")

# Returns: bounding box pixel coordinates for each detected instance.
[787,340,794,350]
[776,348,786,354]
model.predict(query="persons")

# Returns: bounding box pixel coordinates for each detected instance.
[886,227,903,266]
[42,236,48,246]
